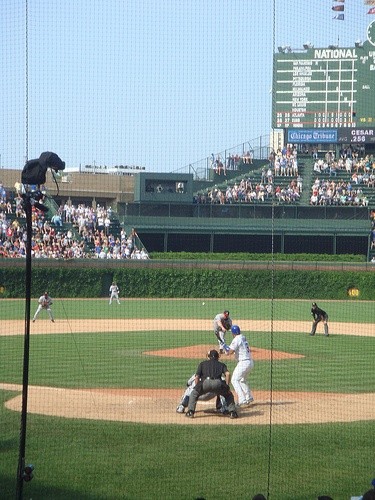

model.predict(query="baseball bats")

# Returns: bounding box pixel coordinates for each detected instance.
[215,331,224,344]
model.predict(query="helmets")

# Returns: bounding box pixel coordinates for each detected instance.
[231,325,240,335]
[208,350,219,359]
[224,311,230,316]
[312,302,316,306]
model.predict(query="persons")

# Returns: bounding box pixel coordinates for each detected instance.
[32,292,54,323]
[177,350,238,419]
[225,325,254,406]
[310,302,329,336]
[0,181,149,259]
[197,143,375,209]
[370,210,375,262]
[214,311,232,355]
[149,183,167,193]
[109,282,120,305]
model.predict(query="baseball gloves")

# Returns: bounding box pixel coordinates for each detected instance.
[42,302,49,308]
[225,323,231,330]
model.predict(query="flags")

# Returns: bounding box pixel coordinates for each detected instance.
[332,0,344,20]
[363,0,375,14]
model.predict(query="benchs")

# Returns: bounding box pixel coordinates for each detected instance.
[194,158,375,210]
[0,199,121,254]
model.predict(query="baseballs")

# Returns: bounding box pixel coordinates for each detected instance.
[202,303,205,305]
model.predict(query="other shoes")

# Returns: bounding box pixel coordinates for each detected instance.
[185,410,195,418]
[229,410,238,418]
[247,396,254,404]
[222,410,228,415]
[236,399,248,407]
[176,405,184,413]
